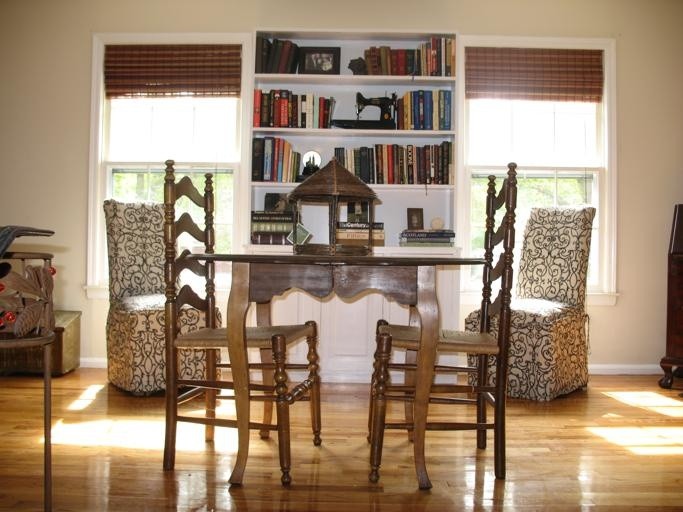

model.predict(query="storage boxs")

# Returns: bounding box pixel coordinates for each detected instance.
[0,311,81,376]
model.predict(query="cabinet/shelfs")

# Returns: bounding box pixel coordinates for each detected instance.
[246,26,462,248]
[248,251,450,375]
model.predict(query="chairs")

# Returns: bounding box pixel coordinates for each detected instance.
[102,198,221,398]
[161,159,321,484]
[464,207,596,404]
[0,252,55,480]
[367,162,517,486]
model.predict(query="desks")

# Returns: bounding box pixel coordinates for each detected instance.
[184,250,492,490]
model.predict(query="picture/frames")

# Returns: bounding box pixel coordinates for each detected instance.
[298,45,341,77]
[406,207,424,231]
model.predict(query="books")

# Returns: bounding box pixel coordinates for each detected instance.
[364,36,456,77]
[256,36,299,74]
[252,136,301,183]
[334,140,453,185]
[400,229,455,245]
[397,90,452,131]
[335,198,385,246]
[251,192,313,245]
[253,89,336,128]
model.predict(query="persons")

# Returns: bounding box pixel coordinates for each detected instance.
[308,54,319,69]
[321,57,332,71]
